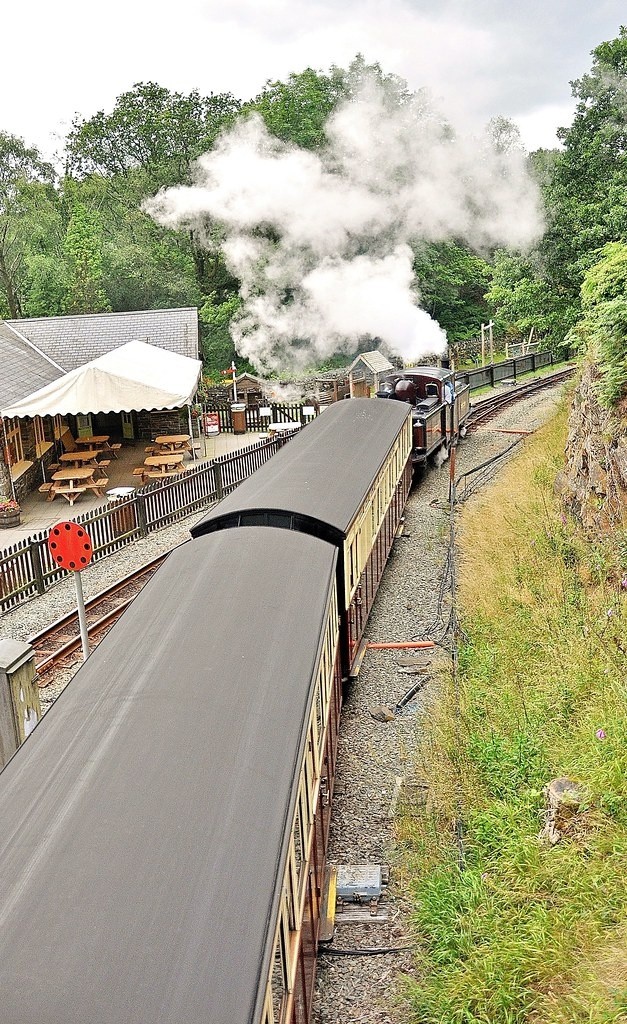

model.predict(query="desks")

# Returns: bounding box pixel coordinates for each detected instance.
[59,451,108,478]
[141,455,189,482]
[148,435,199,460]
[75,436,120,460]
[267,422,302,442]
[44,468,104,502]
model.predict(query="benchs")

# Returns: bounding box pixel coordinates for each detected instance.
[37,439,202,495]
[259,432,269,439]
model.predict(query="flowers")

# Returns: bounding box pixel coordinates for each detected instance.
[0,494,23,512]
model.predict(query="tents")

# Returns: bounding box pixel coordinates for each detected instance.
[0,340,203,502]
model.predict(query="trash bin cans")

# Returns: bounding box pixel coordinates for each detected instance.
[106,488,138,542]
[230,403,248,435]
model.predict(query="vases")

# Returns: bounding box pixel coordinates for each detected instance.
[0,511,21,529]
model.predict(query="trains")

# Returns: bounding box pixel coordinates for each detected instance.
[1,356,471,1024]
